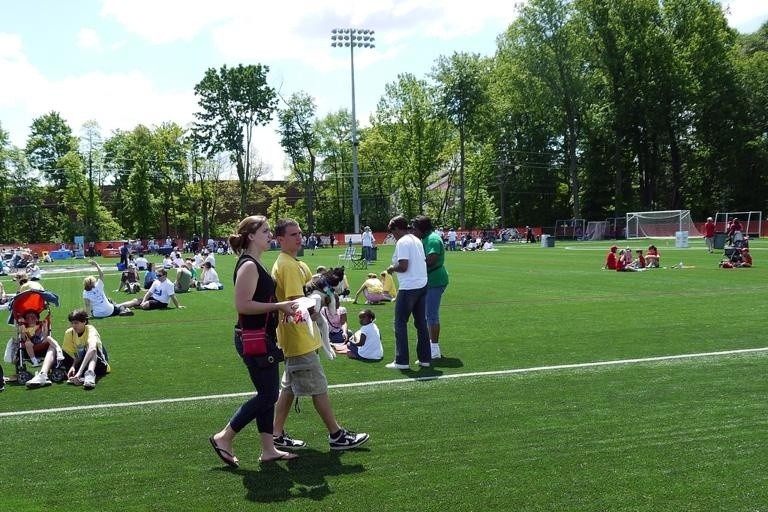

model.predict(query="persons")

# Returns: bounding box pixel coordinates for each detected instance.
[317,266,396,362]
[412,215,449,359]
[81,259,186,318]
[601,245,661,271]
[206,237,241,255]
[435,225,537,252]
[271,217,370,450]
[84,240,113,257]
[1,280,111,388]
[116,235,223,293]
[1,247,54,286]
[348,226,376,259]
[209,215,320,468]
[270,233,334,255]
[384,216,431,369]
[704,216,752,269]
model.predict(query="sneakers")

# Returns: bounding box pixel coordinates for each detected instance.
[31,357,38,367]
[119,309,133,316]
[430,342,440,359]
[272,429,307,451]
[414,359,430,367]
[25,370,52,389]
[83,370,96,388]
[327,426,370,450]
[385,360,409,370]
[56,348,65,361]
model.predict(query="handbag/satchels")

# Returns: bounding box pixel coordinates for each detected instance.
[241,326,267,357]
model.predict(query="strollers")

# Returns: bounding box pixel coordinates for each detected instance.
[460,235,479,251]
[6,289,68,384]
[717,231,754,269]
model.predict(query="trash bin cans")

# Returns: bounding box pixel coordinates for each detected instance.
[714,231,725,249]
[546,236,555,247]
[368,247,377,260]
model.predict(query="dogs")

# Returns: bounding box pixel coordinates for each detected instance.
[303,266,344,361]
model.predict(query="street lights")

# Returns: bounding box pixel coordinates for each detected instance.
[329,26,380,231]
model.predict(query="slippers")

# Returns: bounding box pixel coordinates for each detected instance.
[258,451,299,461]
[208,432,240,468]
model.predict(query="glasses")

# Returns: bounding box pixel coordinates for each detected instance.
[156,274,164,277]
